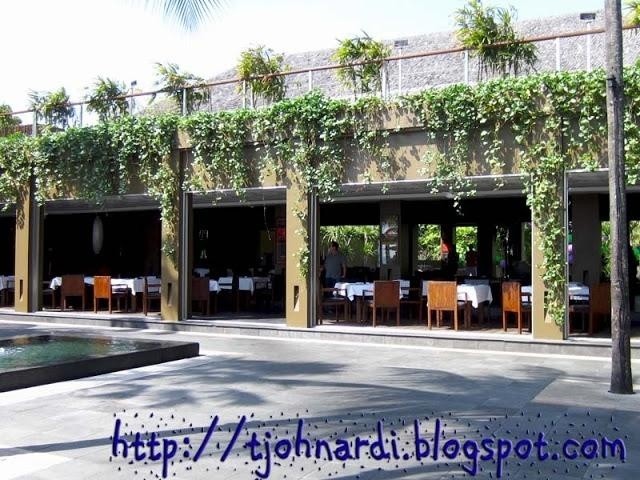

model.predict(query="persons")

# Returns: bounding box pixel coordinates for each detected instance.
[464,243,479,277]
[319,240,347,316]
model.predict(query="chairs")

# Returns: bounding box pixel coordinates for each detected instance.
[315,277,612,335]
[0,274,274,317]
